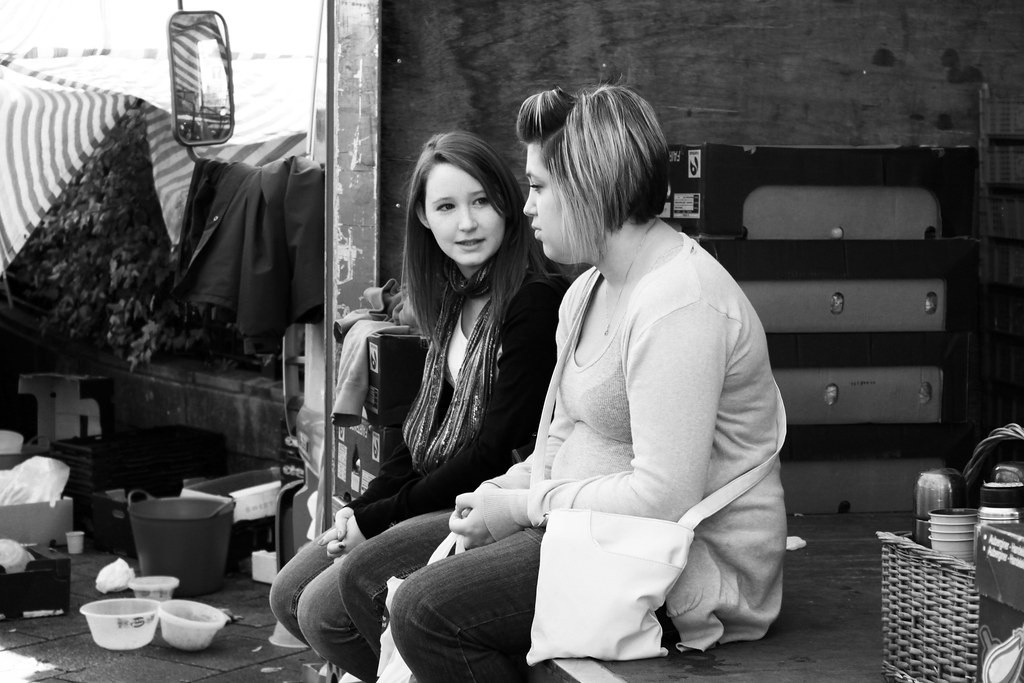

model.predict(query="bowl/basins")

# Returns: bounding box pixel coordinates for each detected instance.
[80,598,161,650]
[0,430,24,455]
[159,600,231,650]
[0,539,36,574]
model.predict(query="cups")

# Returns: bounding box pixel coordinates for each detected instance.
[66,531,85,554]
[928,509,977,563]
[129,576,180,628]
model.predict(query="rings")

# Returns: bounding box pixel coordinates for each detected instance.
[336,541,346,555]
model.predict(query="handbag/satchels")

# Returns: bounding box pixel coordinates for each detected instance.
[526,508,695,665]
[375,530,470,682]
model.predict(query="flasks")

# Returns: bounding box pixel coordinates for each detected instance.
[912,467,967,548]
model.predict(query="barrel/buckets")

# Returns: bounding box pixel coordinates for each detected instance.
[127,489,234,597]
[979,484,1024,519]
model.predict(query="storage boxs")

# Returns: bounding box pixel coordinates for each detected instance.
[0,425,275,620]
[323,329,431,529]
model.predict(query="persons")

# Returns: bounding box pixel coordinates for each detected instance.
[341,84,788,683]
[269,130,573,682]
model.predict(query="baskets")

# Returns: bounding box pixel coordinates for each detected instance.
[875,424,1024,683]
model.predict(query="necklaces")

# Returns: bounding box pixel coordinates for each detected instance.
[601,221,648,336]
